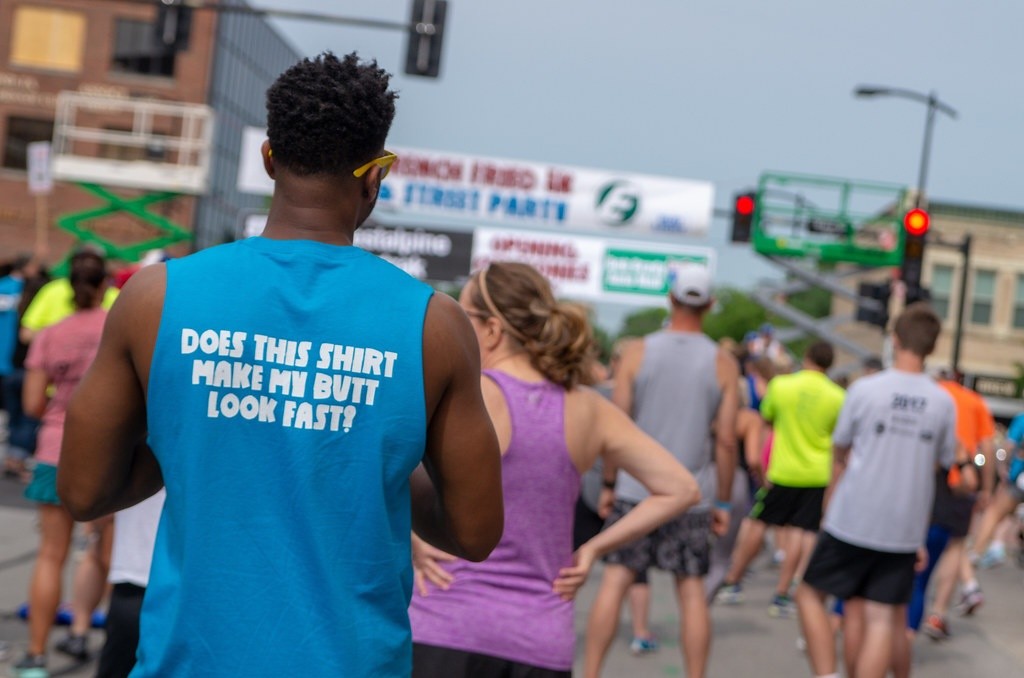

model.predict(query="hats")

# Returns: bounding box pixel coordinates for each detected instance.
[668,262,711,305]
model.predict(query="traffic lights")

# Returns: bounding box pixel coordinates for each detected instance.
[901,209,931,296]
[732,191,756,243]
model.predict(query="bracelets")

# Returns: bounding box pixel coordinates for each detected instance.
[601,479,616,490]
[716,500,730,510]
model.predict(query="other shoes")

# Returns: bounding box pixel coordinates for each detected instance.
[718,584,742,603]
[55,633,90,663]
[952,585,984,618]
[924,614,948,642]
[769,592,795,618]
[978,544,1006,570]
[13,651,48,678]
[630,638,658,654]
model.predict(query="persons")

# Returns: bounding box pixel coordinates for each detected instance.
[710,326,1024,678]
[56,53,505,678]
[584,271,740,677]
[1,249,169,678]
[797,308,957,678]
[569,334,657,655]
[406,263,700,678]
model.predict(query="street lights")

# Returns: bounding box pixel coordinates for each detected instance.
[852,85,958,209]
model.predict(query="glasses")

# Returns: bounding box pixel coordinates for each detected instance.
[268,147,398,180]
